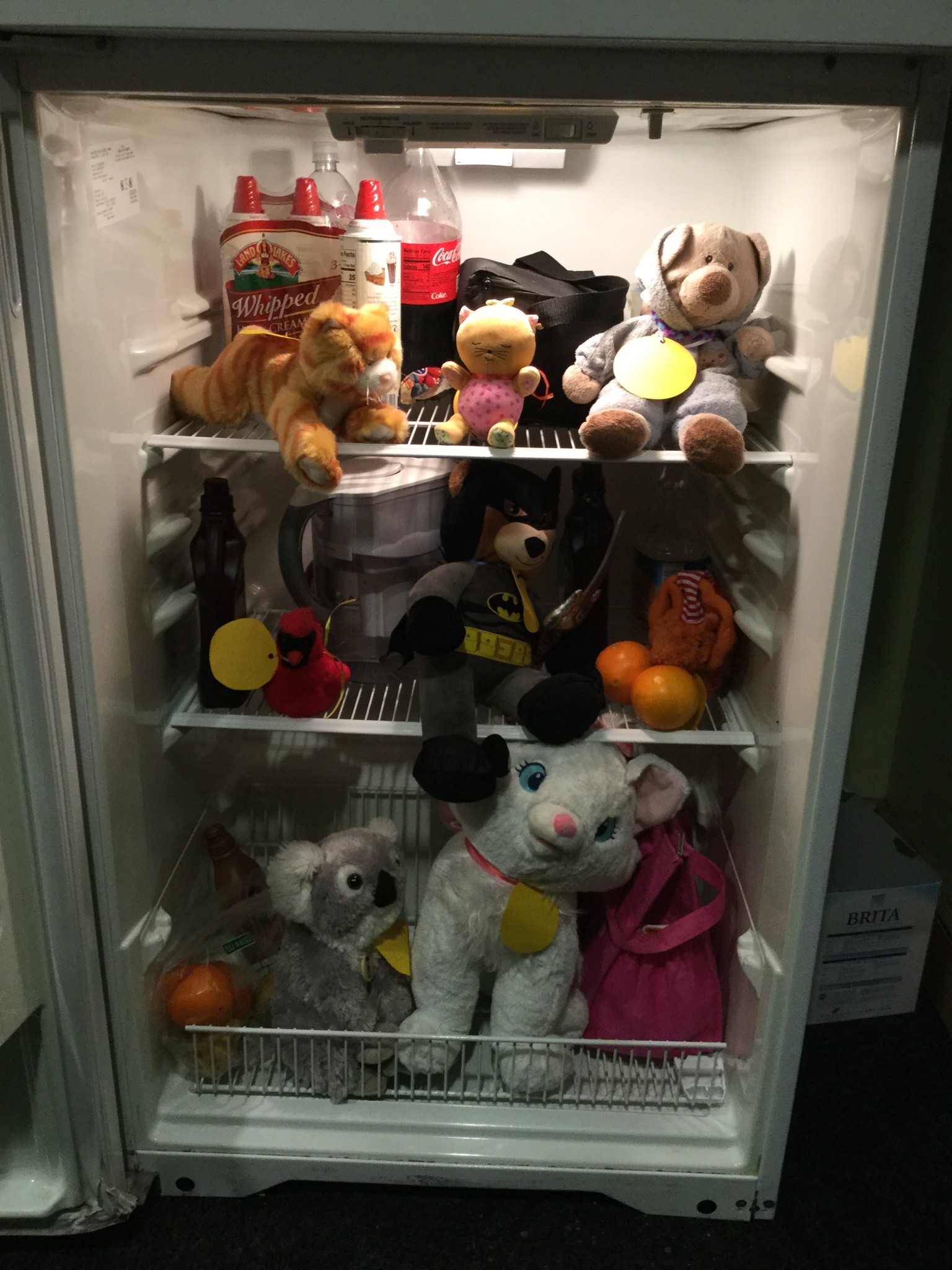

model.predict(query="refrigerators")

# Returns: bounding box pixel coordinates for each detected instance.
[1,2,952,1240]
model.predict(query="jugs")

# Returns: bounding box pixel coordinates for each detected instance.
[276,457,458,685]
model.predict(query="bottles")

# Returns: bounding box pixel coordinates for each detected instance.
[221,175,271,345]
[308,139,357,226]
[288,177,326,229]
[203,821,285,964]
[189,477,250,710]
[379,139,462,402]
[339,180,400,408]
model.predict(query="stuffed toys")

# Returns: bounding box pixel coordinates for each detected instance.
[262,608,351,719]
[434,297,540,449]
[169,298,412,494]
[562,222,775,475]
[649,568,735,673]
[383,458,603,805]
[268,817,414,1103]
[397,714,692,1092]
